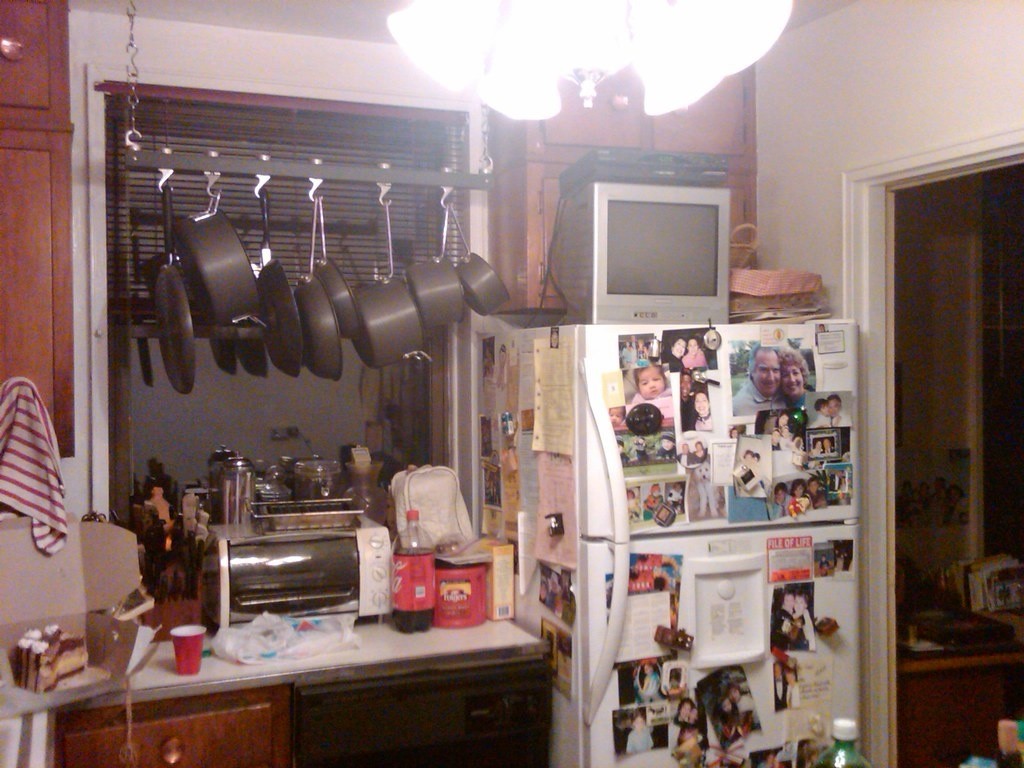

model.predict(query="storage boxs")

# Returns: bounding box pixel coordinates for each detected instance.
[0,517,164,718]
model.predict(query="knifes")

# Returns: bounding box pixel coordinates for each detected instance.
[133,487,199,589]
[139,457,180,511]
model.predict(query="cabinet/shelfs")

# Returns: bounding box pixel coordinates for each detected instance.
[55,682,294,768]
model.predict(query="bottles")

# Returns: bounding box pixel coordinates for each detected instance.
[389,511,436,634]
[812,719,875,768]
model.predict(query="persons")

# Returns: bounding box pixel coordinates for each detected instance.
[605,331,855,768]
[896,478,968,528]
[539,568,577,630]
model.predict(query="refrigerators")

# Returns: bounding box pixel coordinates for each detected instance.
[467,301,866,768]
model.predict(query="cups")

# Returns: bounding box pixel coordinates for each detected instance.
[170,624,206,677]
[345,460,403,509]
[85,609,139,677]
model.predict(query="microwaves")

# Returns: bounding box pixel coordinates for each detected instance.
[200,519,394,630]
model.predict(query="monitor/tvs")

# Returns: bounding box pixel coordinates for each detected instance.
[556,182,730,325]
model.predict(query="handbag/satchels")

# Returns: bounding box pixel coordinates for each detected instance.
[391,464,473,546]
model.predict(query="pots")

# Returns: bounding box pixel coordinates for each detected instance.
[145,162,511,395]
